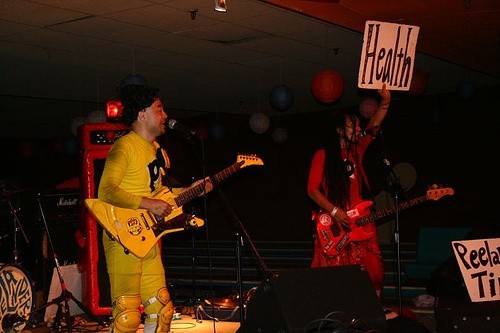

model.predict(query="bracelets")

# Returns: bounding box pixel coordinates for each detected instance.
[379,102,390,105]
[378,105,389,109]
[331,206,339,217]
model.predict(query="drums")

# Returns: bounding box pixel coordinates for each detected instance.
[0,264,36,333]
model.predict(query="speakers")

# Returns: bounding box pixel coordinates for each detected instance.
[235,263,387,333]
[23,218,81,309]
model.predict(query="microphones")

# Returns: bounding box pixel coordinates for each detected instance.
[168,114,200,129]
[9,314,28,325]
[358,125,378,139]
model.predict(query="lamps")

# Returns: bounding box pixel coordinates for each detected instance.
[121,48,145,101]
[311,25,345,102]
[267,58,295,112]
[72,70,106,137]
[273,112,288,143]
[407,68,428,97]
[249,90,270,134]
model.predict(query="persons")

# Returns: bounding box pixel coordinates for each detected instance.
[97,85,214,333]
[307,84,391,304]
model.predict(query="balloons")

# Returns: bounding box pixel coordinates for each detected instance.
[359,97,378,119]
[311,69,343,103]
[270,85,293,112]
[406,69,427,98]
[272,126,290,142]
[250,112,270,132]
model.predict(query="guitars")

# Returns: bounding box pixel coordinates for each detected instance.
[317,185,455,258]
[85,151,264,258]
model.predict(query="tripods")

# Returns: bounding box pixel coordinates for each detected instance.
[21,193,106,333]
